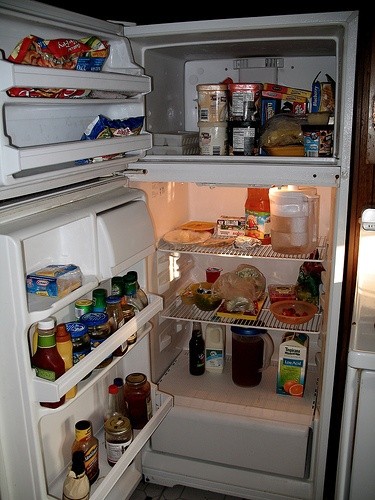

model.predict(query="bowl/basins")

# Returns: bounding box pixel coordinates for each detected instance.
[189,281,223,312]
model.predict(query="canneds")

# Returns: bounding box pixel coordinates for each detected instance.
[64,304,137,382]
[123,373,153,430]
[104,416,134,467]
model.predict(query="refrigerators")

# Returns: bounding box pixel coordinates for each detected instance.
[0,0,358,500]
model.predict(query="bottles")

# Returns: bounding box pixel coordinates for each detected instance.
[71,420,100,486]
[32,318,66,409]
[62,451,90,500]
[103,386,121,448]
[123,373,152,430]
[189,322,205,377]
[92,269,148,356]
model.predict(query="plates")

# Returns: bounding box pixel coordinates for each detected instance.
[270,301,318,324]
[163,230,212,245]
[178,220,216,233]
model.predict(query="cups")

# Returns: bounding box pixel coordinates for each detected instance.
[230,326,274,387]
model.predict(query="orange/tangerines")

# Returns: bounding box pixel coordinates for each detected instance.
[284,380,304,396]
